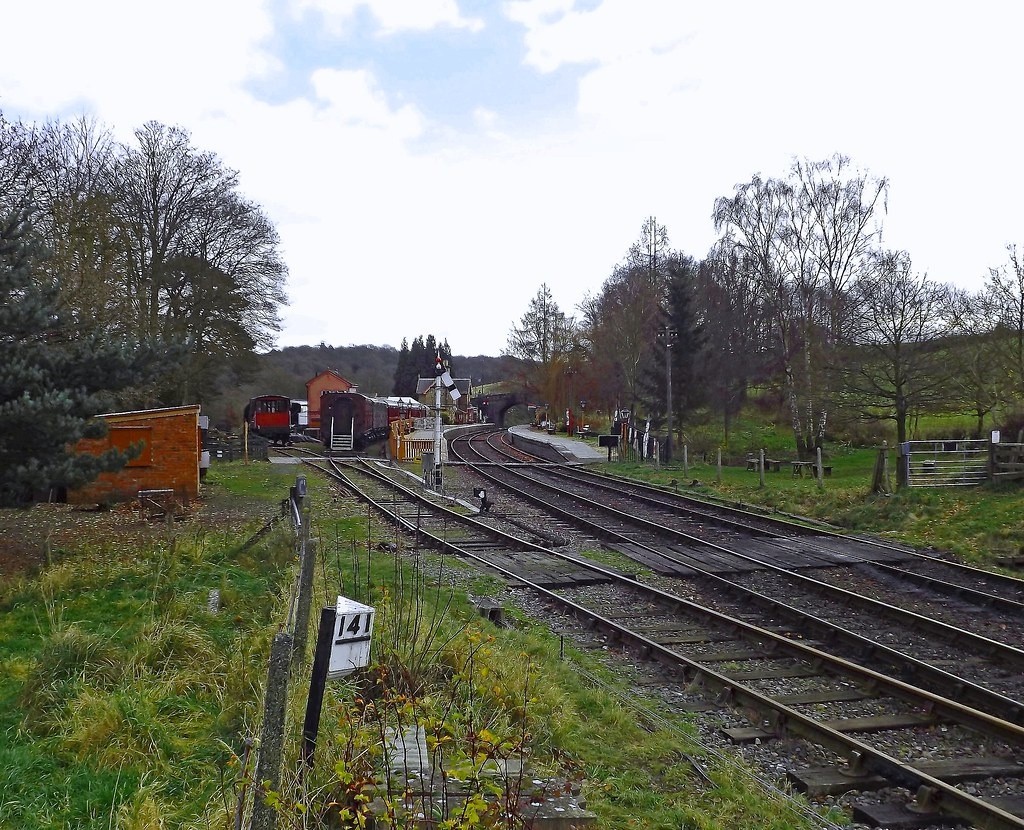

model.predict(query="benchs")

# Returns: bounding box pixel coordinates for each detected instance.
[746,459,760,471]
[813,465,832,476]
[923,460,935,472]
[765,458,780,472]
[547,429,555,434]
[577,432,588,439]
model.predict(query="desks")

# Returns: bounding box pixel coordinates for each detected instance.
[792,461,814,479]
[138,490,173,520]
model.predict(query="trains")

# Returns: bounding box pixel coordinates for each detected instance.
[249,395,292,448]
[318,389,428,459]
